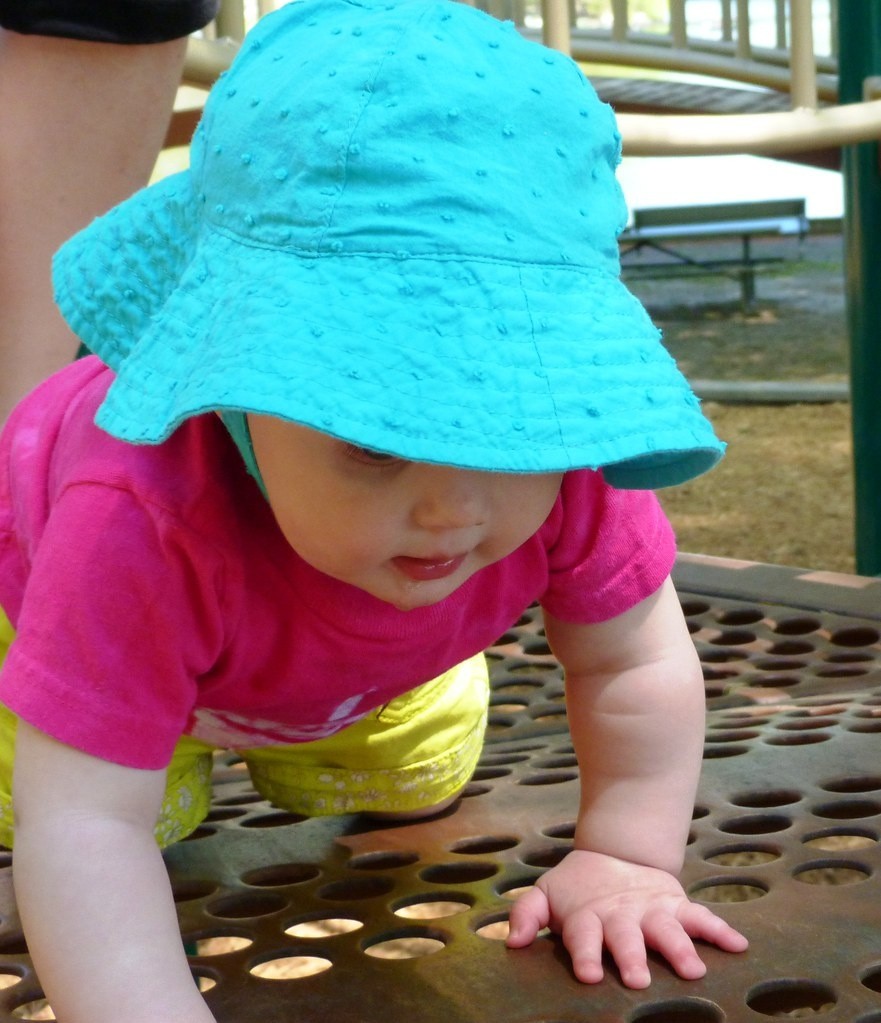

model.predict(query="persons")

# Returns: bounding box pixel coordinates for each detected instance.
[0,0,750,1023]
[1,1,226,430]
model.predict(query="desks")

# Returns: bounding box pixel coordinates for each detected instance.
[616,154,806,315]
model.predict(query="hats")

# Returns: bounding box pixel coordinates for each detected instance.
[51,0,723,489]
